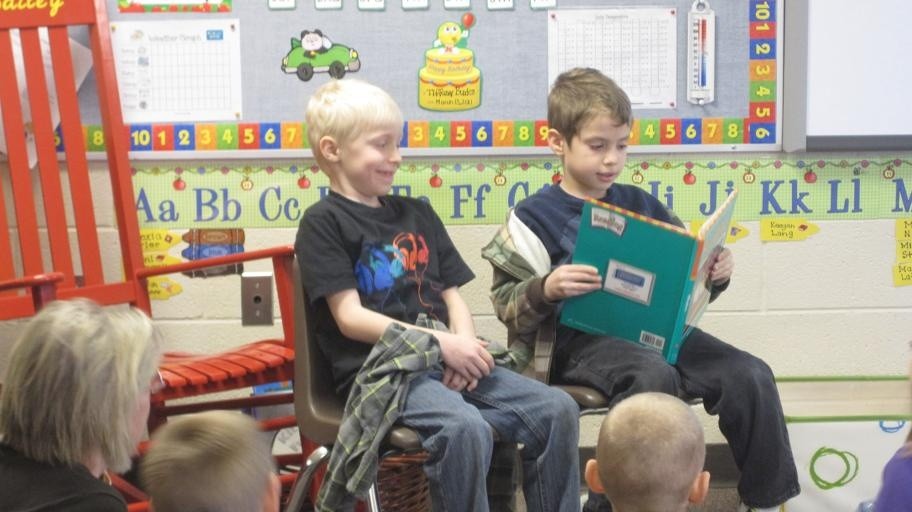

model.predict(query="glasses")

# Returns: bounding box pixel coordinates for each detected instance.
[147,369,166,395]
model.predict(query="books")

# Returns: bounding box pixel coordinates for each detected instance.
[560,189,739,365]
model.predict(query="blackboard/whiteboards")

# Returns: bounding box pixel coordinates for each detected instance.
[783,0,912,154]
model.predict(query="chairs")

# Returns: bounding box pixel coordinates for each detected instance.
[289,254,540,512]
[0,1,329,512]
[506,306,705,414]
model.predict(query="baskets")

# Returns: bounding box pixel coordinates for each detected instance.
[374,452,432,512]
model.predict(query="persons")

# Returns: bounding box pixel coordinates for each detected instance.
[0,298,162,512]
[141,409,281,512]
[585,392,711,512]
[482,69,801,512]
[294,80,581,512]
